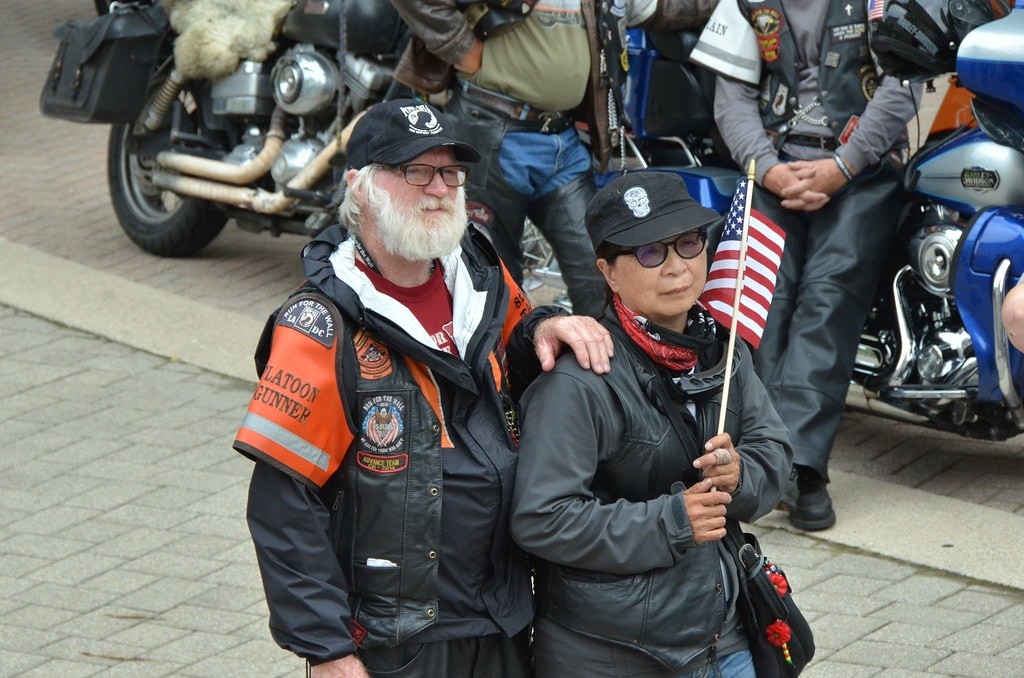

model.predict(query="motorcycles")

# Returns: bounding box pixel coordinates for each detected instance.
[38,0,1024,443]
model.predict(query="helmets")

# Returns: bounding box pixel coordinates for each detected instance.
[868,0,993,88]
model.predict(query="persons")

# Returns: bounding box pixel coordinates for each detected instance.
[510,170,795,678]
[231,100,615,678]
[390,0,1024,534]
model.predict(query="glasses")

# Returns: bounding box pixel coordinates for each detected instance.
[607,231,707,268]
[369,163,471,187]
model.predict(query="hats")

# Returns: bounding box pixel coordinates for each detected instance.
[584,172,720,255]
[345,98,482,171]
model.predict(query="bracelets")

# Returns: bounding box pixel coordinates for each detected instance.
[831,151,852,182]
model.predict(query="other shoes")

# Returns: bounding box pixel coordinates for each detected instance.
[789,466,835,530]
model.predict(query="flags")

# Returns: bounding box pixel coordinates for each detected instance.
[702,177,787,349]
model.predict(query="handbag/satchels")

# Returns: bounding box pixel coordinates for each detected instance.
[739,532,815,678]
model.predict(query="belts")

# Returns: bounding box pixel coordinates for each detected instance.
[459,84,572,134]
[784,135,836,150]
